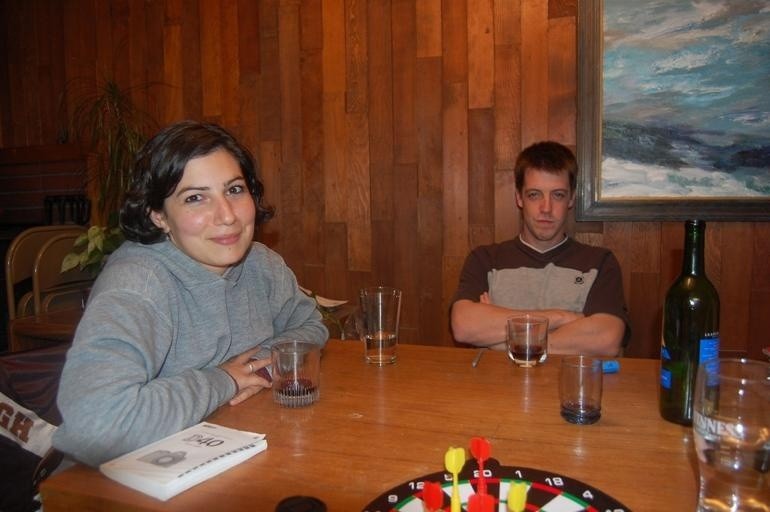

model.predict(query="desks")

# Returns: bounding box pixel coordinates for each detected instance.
[8,298,359,354]
[40,339,769,511]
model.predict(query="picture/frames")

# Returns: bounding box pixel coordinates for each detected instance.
[575,1,770,222]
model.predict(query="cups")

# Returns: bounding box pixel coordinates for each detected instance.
[359,286,403,367]
[506,316,549,368]
[560,355,603,425]
[271,341,322,408]
[693,359,767,512]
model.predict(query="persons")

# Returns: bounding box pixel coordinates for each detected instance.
[31,120,329,512]
[447,139,635,360]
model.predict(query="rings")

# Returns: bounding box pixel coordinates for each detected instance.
[246,361,253,374]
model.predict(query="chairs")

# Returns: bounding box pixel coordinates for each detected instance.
[0,340,71,425]
[5,225,90,353]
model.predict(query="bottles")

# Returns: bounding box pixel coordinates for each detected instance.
[660,218,721,426]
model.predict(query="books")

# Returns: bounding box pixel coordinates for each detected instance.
[100,420,267,502]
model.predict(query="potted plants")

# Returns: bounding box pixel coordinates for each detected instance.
[60,225,124,316]
